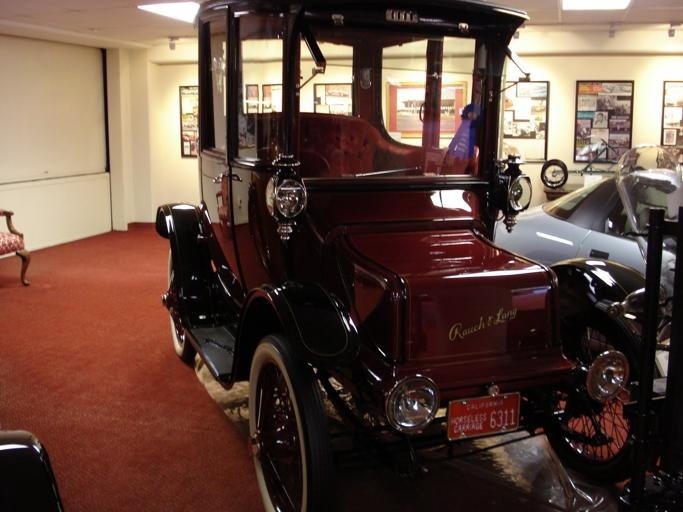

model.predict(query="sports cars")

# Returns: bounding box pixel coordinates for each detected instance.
[541,141,683,190]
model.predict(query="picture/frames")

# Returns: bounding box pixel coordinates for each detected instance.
[572,79,635,163]
[497,80,549,163]
[244,83,259,113]
[384,81,468,132]
[261,83,282,113]
[660,80,683,147]
[312,83,354,117]
[179,84,201,159]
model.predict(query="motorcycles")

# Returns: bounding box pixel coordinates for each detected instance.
[500,151,524,202]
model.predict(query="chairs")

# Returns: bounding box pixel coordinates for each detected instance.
[0,207,30,287]
[266,110,379,180]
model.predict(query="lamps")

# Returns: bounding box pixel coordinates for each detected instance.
[511,22,678,39]
[166,33,196,52]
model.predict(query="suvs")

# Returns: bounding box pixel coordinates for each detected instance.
[144,0,649,511]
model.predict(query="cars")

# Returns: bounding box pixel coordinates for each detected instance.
[576,143,607,160]
[486,168,683,306]
[0,427,64,512]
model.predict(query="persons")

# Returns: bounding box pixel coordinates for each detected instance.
[595,113,607,128]
